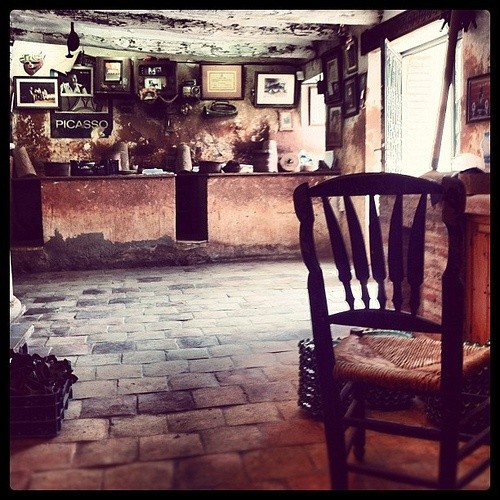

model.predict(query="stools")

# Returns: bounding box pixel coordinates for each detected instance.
[10,325,35,357]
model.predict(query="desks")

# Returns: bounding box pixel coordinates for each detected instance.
[11,174,178,247]
[176,172,343,240]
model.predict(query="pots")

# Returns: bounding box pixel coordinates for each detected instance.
[44,161,69,177]
[198,160,221,173]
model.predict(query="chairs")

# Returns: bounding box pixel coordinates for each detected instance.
[291,172,490,490]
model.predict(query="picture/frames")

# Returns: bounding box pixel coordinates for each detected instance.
[254,71,298,108]
[277,111,294,132]
[54,66,93,97]
[103,59,123,85]
[466,72,490,125]
[323,32,359,151]
[13,76,62,111]
[199,61,244,100]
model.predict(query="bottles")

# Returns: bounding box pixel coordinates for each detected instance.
[481,132,490,173]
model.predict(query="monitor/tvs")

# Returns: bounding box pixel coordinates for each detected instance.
[96,56,130,92]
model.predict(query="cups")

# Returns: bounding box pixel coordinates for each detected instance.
[107,159,119,175]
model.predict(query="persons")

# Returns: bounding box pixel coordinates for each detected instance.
[345,85,355,109]
[477,84,485,109]
[27,87,47,103]
[328,111,340,146]
[149,80,158,87]
[328,81,339,101]
[60,71,88,94]
